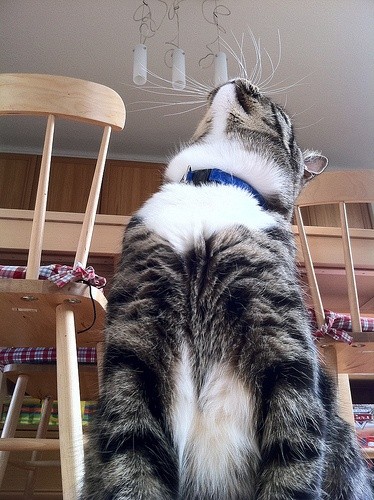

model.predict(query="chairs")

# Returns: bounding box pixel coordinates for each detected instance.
[0,344,100,500]
[288,168,374,460]
[0,72,125,500]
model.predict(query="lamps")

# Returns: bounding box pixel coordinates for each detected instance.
[132,43,148,86]
[212,53,228,87]
[171,48,186,92]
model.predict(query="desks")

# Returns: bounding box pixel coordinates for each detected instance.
[0,207,374,373]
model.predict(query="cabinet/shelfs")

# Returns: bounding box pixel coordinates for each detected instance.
[292,202,374,229]
[0,152,167,216]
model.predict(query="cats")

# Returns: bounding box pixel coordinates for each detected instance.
[80,31,374,500]
